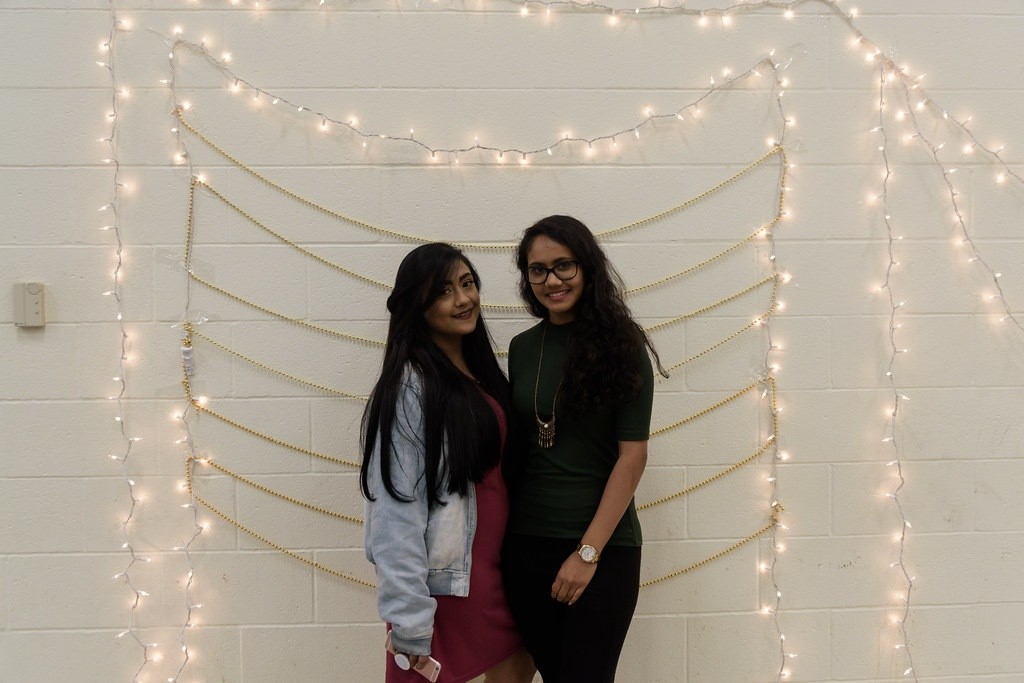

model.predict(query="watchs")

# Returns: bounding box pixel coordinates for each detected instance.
[575,541,601,567]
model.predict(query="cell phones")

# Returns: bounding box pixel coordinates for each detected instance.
[385,629,441,683]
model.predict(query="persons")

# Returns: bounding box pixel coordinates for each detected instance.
[355,237,540,683]
[497,214,654,683]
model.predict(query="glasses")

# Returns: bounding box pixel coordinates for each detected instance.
[524,259,579,284]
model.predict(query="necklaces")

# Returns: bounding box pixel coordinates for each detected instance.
[531,317,586,448]
